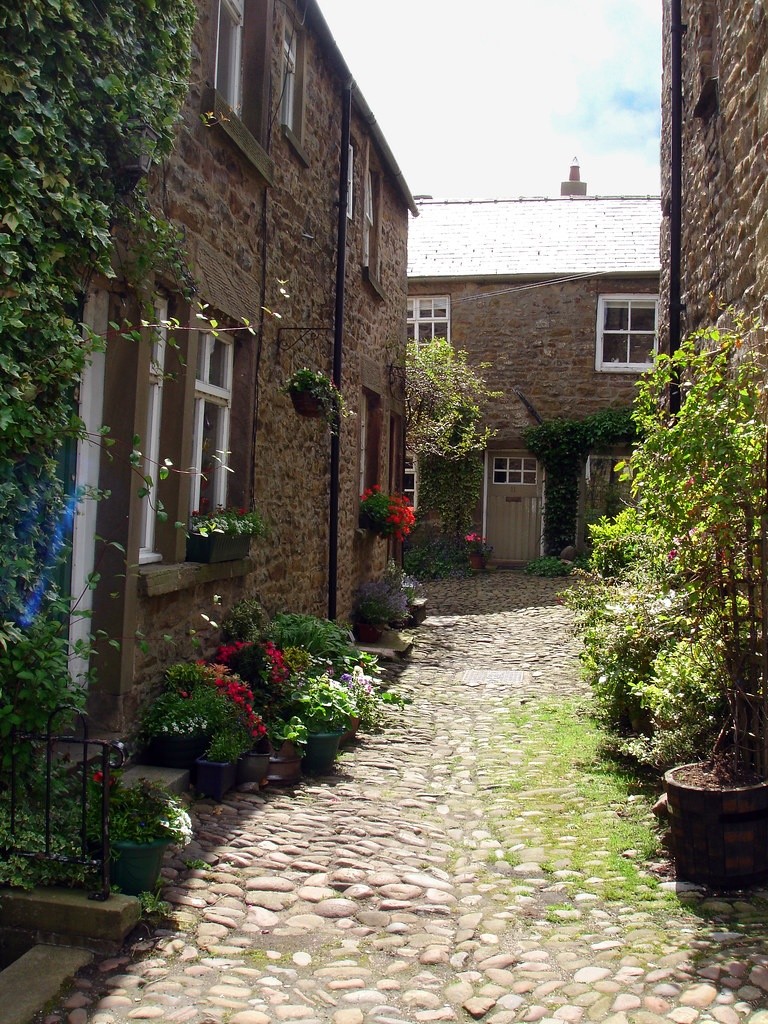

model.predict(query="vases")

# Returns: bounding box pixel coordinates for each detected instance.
[408,596,428,626]
[87,835,174,895]
[135,717,359,803]
[355,619,383,643]
[186,534,249,564]
[469,554,486,570]
[359,513,370,530]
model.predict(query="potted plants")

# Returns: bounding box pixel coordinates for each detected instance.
[279,366,357,437]
[612,309,768,891]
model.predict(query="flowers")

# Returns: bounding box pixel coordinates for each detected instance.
[355,558,419,636]
[359,485,415,544]
[68,770,193,849]
[187,497,269,540]
[465,533,487,555]
[141,599,414,764]
